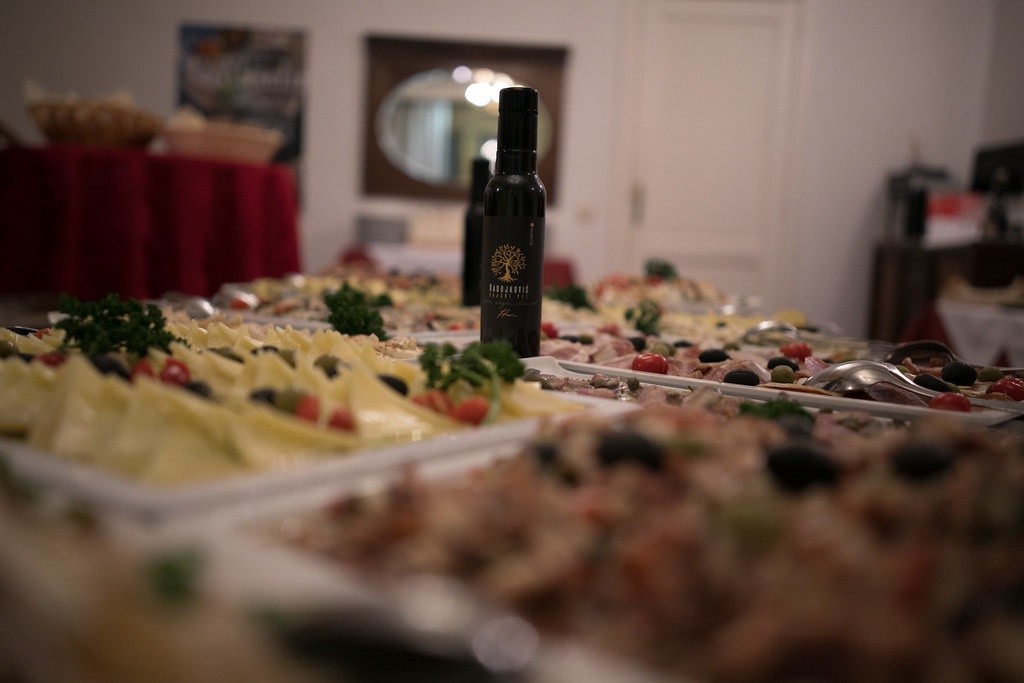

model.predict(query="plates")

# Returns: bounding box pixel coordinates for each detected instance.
[0,314,1024,683]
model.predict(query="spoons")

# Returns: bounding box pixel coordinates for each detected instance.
[739,319,888,346]
[799,361,1024,415]
[884,340,1024,374]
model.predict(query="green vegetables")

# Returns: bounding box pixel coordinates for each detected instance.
[52,256,848,496]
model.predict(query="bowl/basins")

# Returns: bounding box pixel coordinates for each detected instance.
[160,119,285,166]
[26,99,164,151]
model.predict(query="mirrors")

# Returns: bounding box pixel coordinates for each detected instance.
[363,33,570,202]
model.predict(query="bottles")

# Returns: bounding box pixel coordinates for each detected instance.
[463,157,493,306]
[480,85,546,358]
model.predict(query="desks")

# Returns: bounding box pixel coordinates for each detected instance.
[0,141,302,300]
